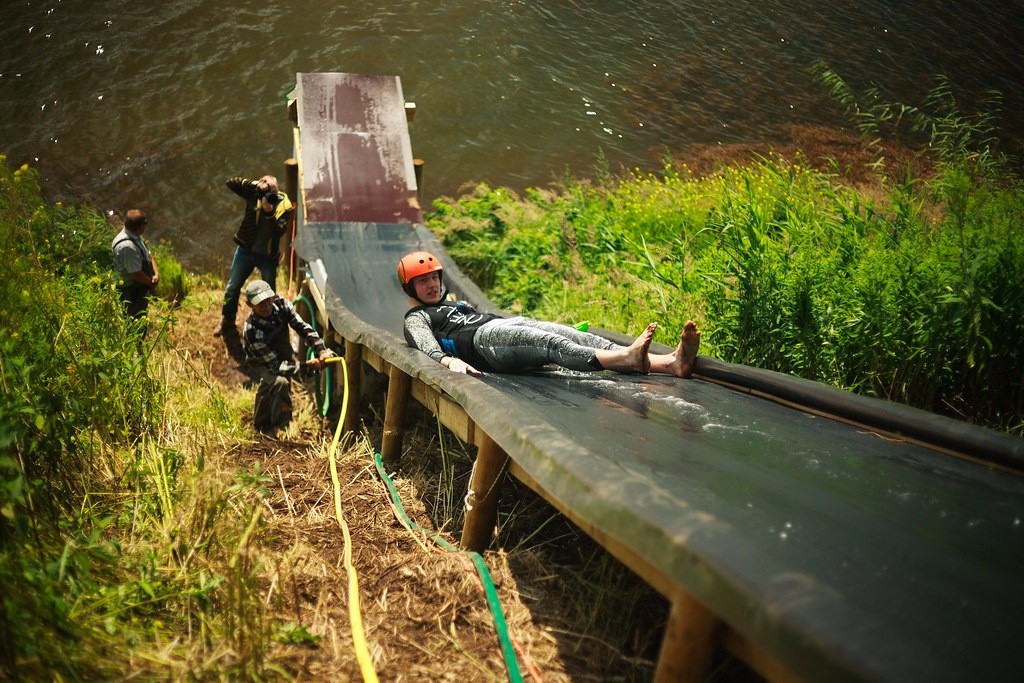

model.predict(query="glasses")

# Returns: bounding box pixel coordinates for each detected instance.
[142,219,148,225]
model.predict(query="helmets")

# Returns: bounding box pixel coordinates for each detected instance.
[398,251,445,295]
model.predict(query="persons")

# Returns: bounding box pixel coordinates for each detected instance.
[396,250,701,380]
[111,209,161,356]
[239,279,334,437]
[212,174,294,339]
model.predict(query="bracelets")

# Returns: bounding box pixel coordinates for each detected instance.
[447,358,456,369]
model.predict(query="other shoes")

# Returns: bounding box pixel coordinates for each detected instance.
[213,315,236,336]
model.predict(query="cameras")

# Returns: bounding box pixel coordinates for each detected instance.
[256,183,278,204]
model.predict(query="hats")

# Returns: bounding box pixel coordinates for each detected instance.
[245,280,276,304]
